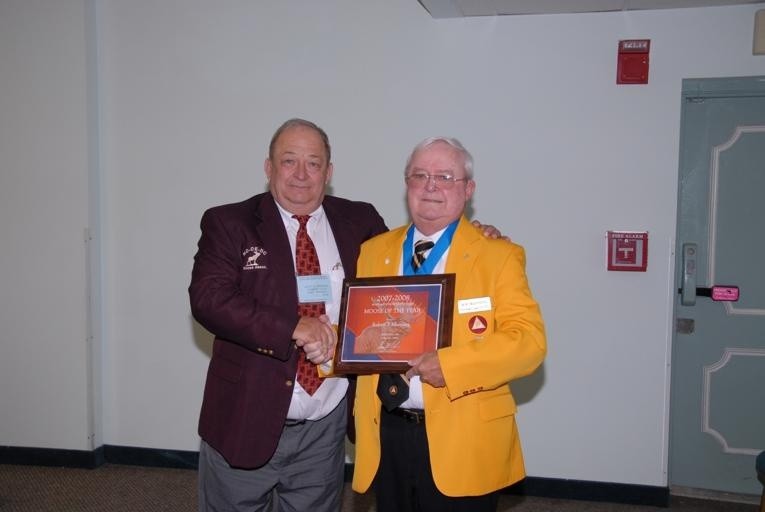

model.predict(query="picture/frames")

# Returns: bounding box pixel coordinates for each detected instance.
[333,272,457,375]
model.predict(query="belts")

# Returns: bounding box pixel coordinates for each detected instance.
[393,409,425,425]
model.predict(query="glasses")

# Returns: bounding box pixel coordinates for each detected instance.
[403,173,466,186]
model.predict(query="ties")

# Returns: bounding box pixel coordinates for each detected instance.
[377,240,433,412]
[292,214,327,397]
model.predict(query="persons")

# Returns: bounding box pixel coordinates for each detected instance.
[187,117,513,512]
[296,134,548,512]
